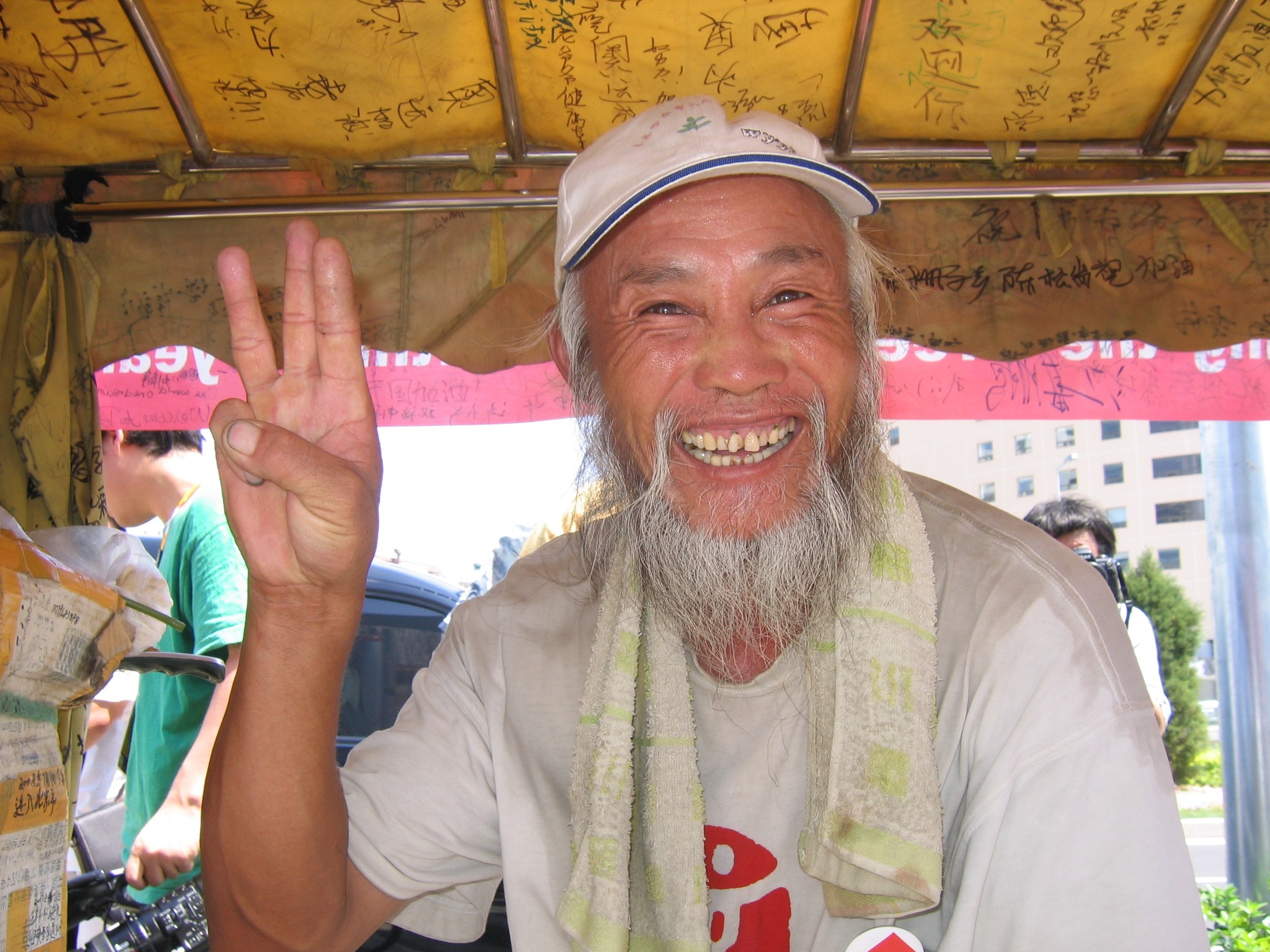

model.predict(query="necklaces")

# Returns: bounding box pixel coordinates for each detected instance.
[156,482,202,566]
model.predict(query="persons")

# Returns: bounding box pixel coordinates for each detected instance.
[1022,499,1172,737]
[100,426,248,910]
[76,519,140,815]
[210,98,1204,951]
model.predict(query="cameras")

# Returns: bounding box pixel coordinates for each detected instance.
[1073,548,1121,604]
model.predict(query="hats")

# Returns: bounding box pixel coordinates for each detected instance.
[548,95,884,300]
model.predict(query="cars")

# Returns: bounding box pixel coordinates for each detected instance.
[135,535,516,952]
